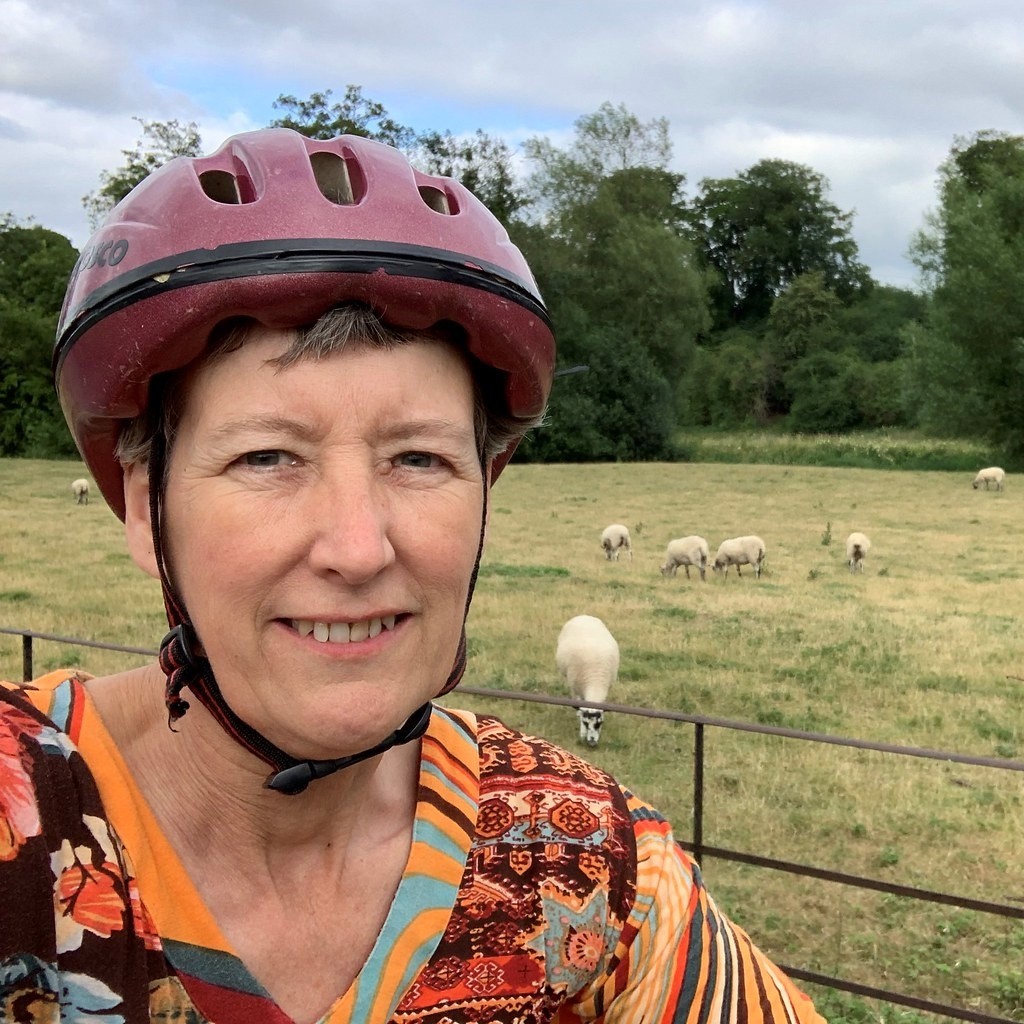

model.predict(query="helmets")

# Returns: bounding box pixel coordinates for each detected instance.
[50,129,556,526]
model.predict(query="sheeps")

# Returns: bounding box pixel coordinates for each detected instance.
[554,613,620,748]
[707,535,766,584]
[70,478,92,506]
[659,535,710,584]
[600,524,633,564]
[846,533,869,577]
[971,466,1006,494]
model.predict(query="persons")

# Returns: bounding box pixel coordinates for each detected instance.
[0,127,832,1024]
